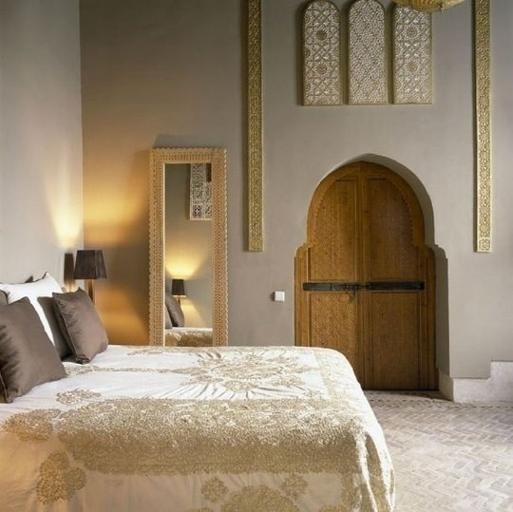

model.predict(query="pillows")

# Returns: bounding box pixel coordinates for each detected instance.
[0,298,66,402]
[0,272,71,361]
[54,284,109,365]
[164,306,172,328]
[165,293,184,328]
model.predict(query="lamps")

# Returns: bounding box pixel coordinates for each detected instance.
[170,278,186,305]
[71,249,108,312]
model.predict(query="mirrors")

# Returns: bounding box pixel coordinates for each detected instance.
[147,147,228,350]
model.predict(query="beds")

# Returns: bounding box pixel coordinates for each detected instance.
[0,343,394,512]
[163,328,212,348]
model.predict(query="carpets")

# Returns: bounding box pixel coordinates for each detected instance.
[362,387,512,511]
